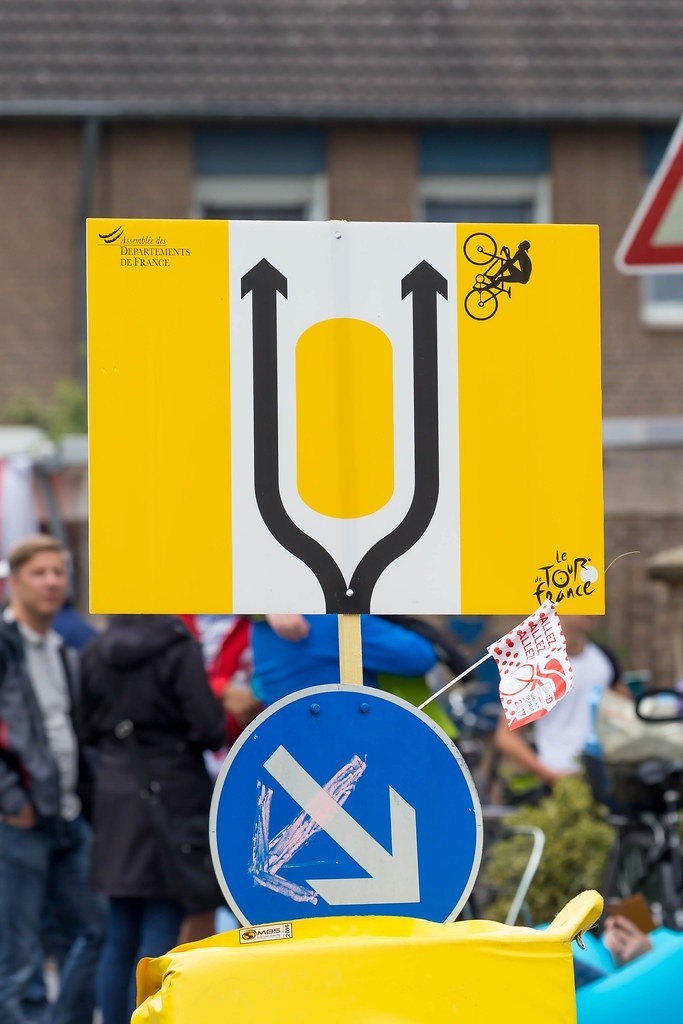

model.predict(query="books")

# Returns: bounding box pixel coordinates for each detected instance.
[608,892,659,934]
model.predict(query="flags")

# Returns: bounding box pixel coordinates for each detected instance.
[486,597,574,731]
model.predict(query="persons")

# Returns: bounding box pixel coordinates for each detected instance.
[176,614,437,783]
[77,614,228,1024]
[604,916,653,966]
[0,535,108,1024]
[495,615,631,807]
